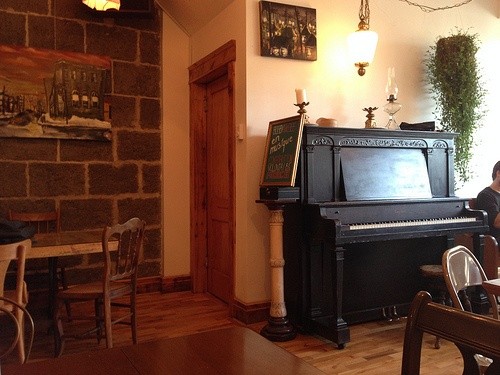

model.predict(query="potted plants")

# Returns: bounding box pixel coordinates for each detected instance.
[423,26,489,183]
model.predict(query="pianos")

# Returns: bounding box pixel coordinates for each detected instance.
[285,124,491,350]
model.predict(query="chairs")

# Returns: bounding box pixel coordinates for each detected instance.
[8,208,71,322]
[443,245,498,319]
[0,239,32,364]
[401,291,500,375]
[53,217,146,359]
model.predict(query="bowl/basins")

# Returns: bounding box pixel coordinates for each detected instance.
[316,117,337,127]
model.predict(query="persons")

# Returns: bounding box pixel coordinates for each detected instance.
[476,161,500,250]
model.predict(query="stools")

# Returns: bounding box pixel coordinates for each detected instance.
[418,265,452,349]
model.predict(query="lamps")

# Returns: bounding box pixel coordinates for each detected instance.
[349,0,378,76]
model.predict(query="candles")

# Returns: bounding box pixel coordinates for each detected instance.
[295,88,306,104]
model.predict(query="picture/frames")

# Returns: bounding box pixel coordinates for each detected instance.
[259,114,305,187]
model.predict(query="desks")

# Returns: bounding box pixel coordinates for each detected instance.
[0,326,330,375]
[30,229,119,336]
